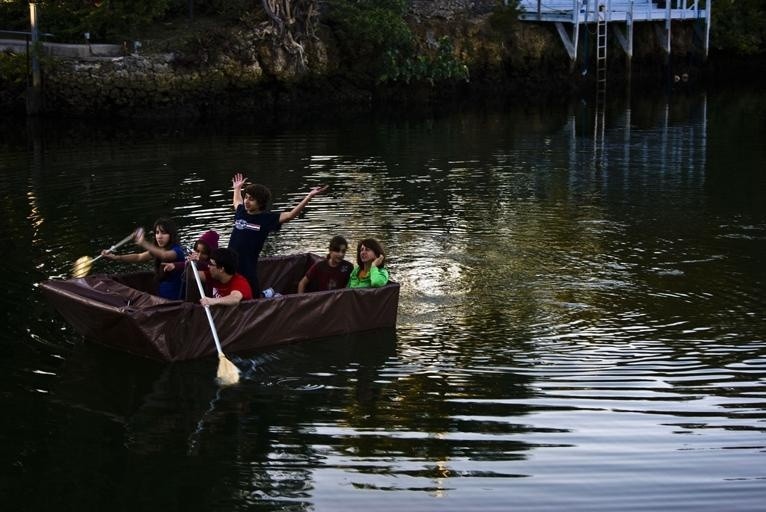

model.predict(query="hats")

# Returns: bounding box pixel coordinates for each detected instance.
[198,231,219,250]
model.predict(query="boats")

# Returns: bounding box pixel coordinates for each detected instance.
[40,252,400,365]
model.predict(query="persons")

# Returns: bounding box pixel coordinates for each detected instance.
[183,248,256,306]
[160,228,219,269]
[225,173,330,299]
[100,215,186,301]
[297,235,355,295]
[344,238,390,288]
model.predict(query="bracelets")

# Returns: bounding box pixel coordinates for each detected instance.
[306,195,311,201]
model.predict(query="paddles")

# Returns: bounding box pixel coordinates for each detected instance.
[75,231,136,279]
[190,260,240,385]
